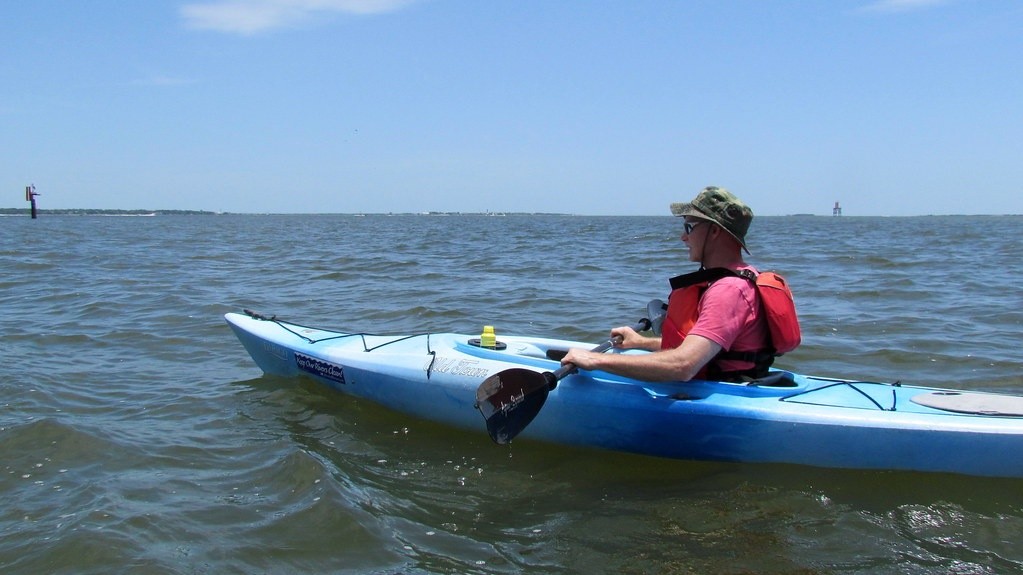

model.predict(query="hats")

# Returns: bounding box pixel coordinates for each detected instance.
[670,185,753,256]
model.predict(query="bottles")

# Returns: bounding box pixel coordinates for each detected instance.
[479,325,497,349]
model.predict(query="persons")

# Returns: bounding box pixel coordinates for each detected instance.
[559,185,774,385]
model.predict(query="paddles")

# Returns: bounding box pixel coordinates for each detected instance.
[475,297,671,446]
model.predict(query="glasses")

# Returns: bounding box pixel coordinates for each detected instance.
[684,221,706,235]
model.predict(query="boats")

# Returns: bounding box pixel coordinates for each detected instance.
[224,310,1023,484]
